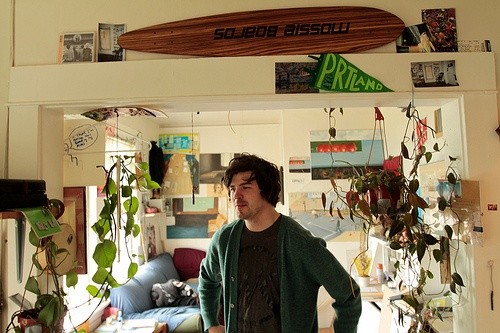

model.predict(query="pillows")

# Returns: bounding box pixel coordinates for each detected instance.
[173,248,206,279]
[148,279,180,307]
[173,280,199,306]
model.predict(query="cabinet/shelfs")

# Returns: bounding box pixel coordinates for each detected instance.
[7,105,64,202]
[352,235,454,333]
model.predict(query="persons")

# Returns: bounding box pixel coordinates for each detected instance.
[198,155,362,333]
[63,43,92,62]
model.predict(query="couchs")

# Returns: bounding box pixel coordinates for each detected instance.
[109,252,203,333]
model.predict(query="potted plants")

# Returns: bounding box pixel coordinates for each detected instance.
[323,105,467,333]
[5,228,79,333]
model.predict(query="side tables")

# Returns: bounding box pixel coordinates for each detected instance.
[95,319,168,333]
